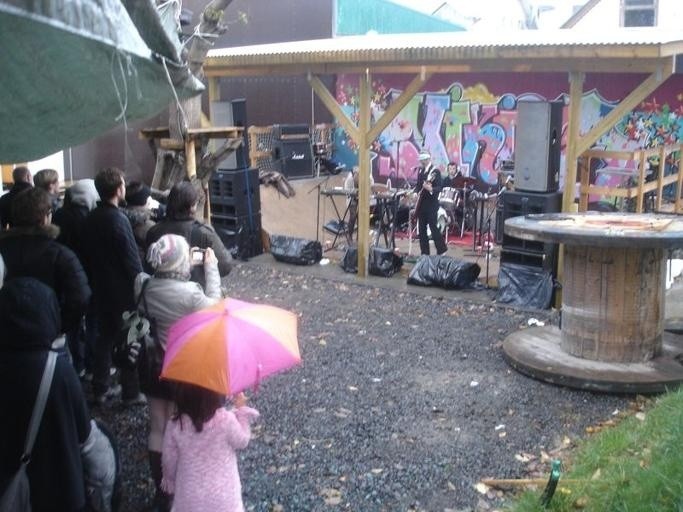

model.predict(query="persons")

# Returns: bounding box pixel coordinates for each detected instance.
[1,277,116,512]
[2,163,233,403]
[441,161,477,236]
[157,377,258,512]
[650,152,678,197]
[411,153,448,258]
[344,164,375,246]
[133,234,235,512]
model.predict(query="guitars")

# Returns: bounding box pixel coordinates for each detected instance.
[413,171,438,218]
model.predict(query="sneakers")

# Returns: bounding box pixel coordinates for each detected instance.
[74,365,147,408]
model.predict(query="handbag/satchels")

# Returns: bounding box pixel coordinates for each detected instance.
[1,471,35,512]
[112,279,163,375]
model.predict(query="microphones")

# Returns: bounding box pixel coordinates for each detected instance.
[505,174,511,184]
[336,164,346,170]
[422,132,426,147]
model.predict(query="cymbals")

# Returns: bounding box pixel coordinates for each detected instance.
[454,177,478,186]
[316,124,338,129]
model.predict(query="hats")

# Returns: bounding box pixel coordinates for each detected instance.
[146,233,190,273]
[416,152,431,161]
[125,180,151,203]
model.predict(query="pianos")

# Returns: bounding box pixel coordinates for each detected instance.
[321,187,406,200]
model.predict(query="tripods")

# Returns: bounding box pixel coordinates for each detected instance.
[476,183,498,291]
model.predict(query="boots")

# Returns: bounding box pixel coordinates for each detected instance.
[147,450,170,512]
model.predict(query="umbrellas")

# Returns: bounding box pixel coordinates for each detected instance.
[157,293,303,400]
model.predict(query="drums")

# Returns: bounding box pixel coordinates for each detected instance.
[446,211,455,233]
[312,142,327,155]
[438,187,461,210]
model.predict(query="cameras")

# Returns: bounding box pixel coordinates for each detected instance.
[192,249,205,265]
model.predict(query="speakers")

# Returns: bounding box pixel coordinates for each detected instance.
[272,139,315,180]
[513,100,564,194]
[501,192,562,310]
[209,98,250,171]
[208,168,263,259]
